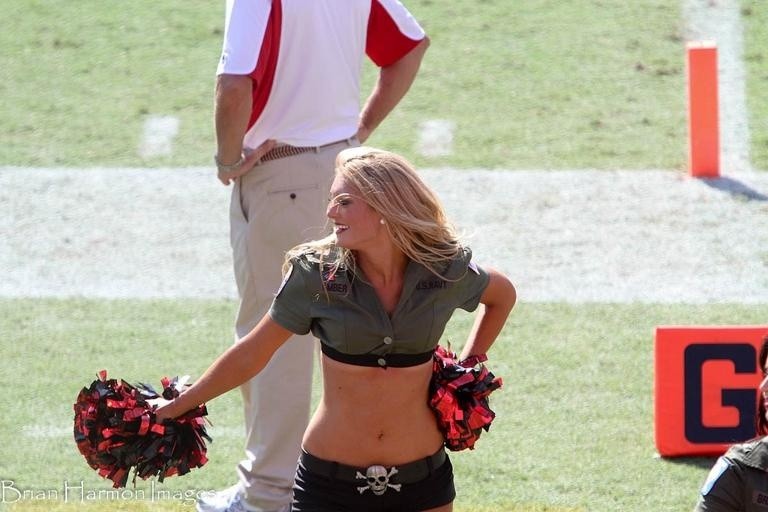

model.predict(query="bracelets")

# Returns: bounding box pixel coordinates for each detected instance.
[214,151,246,173]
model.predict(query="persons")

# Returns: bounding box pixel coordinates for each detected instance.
[132,145,518,510]
[691,330,768,511]
[192,0,430,512]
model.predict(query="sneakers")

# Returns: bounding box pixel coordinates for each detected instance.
[195,483,291,512]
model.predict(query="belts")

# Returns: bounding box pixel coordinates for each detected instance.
[259,134,358,162]
[299,442,446,496]
